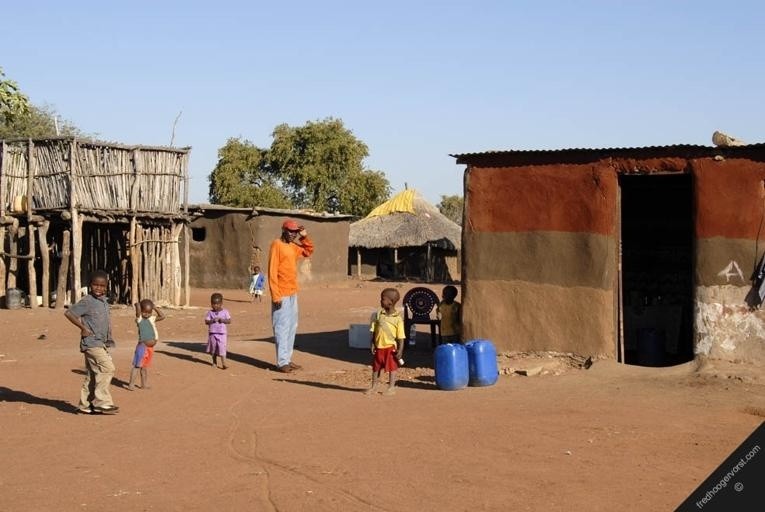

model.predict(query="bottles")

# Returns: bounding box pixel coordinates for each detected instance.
[392,350,406,366]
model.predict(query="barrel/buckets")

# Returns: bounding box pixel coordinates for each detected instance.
[463,337,499,387]
[347,322,373,350]
[432,342,470,391]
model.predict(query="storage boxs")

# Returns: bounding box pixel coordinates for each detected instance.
[349,323,371,349]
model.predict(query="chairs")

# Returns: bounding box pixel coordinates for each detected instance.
[403,286,442,352]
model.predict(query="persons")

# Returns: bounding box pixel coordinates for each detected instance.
[267,220,314,374]
[204,293,231,369]
[128,299,166,391]
[363,288,406,396]
[64,272,121,416]
[249,266,265,303]
[437,286,461,344]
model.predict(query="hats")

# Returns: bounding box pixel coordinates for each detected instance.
[283,220,300,230]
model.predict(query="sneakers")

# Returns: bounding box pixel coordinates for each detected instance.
[289,362,301,368]
[77,407,94,415]
[279,365,295,372]
[94,406,120,413]
